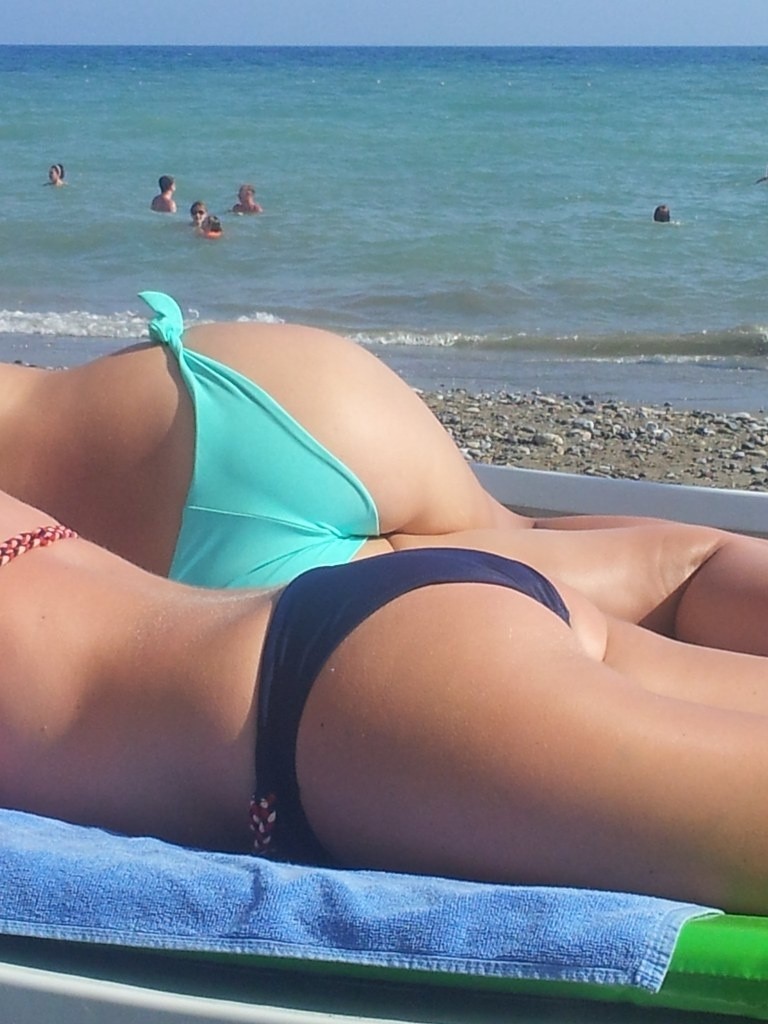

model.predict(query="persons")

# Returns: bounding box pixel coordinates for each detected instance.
[201,217,221,232]
[0,493,767,918]
[43,164,68,186]
[151,175,177,212]
[0,291,767,656]
[233,185,263,214]
[654,204,670,222]
[189,201,207,227]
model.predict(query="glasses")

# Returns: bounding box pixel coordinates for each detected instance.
[192,210,206,216]
